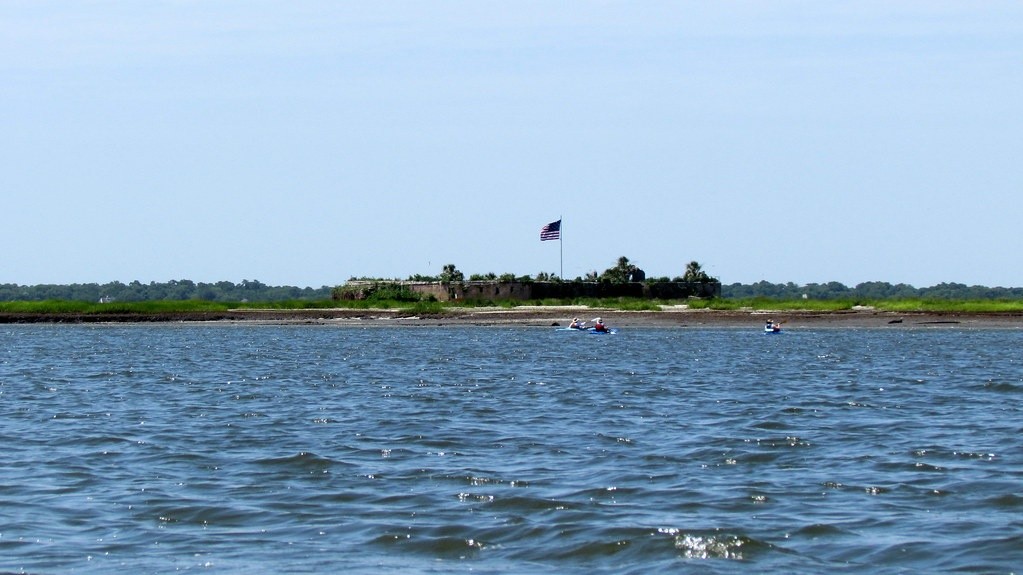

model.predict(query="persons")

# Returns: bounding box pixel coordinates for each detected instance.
[594,320,604,331]
[766,319,780,329]
[569,318,586,328]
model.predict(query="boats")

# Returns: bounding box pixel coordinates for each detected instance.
[555,325,597,332]
[586,325,622,336]
[764,324,775,335]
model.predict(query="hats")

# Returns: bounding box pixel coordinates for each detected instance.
[573,318,579,322]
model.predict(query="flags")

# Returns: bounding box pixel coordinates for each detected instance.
[541,219,561,241]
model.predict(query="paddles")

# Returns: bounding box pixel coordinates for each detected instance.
[776,318,787,326]
[587,317,602,323]
[597,319,617,335]
